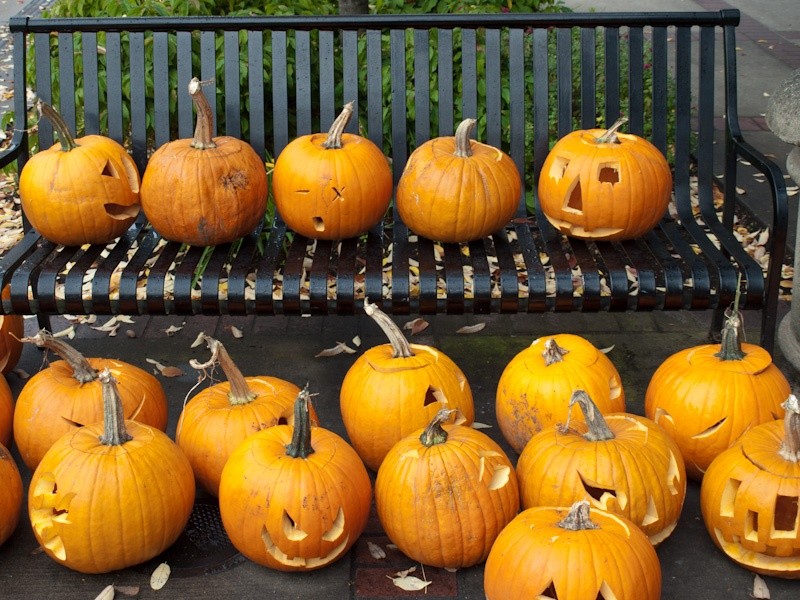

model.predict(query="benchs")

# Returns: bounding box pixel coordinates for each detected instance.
[0,8,791,355]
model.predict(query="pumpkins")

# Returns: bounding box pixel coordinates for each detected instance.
[141,76,270,244]
[397,117,522,244]
[0,455,28,548]
[0,375,15,449]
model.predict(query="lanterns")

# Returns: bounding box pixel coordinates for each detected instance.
[700,394,799,580]
[495,334,626,456]
[644,311,794,482]
[221,388,371,572]
[28,368,196,574]
[19,101,141,245]
[340,297,475,473]
[538,116,673,242]
[7,328,169,473]
[515,390,687,550]
[0,283,25,373]
[484,500,663,600]
[374,408,519,568]
[176,334,318,496]
[271,102,393,240]
